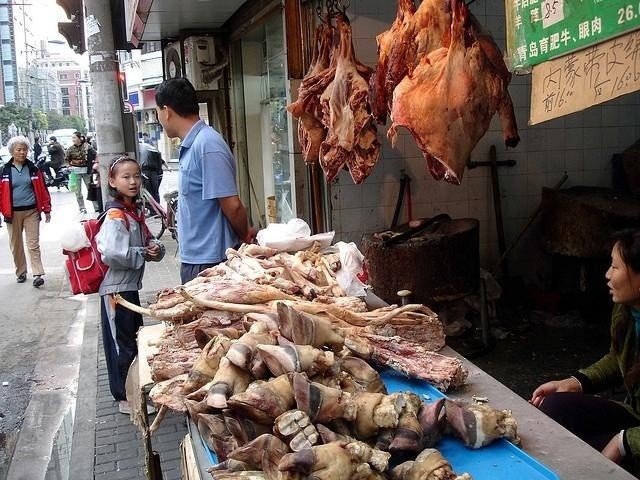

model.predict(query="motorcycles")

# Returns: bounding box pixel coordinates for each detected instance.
[33,154,71,191]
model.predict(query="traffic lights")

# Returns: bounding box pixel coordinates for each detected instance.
[120,72,129,101]
[56,0,86,53]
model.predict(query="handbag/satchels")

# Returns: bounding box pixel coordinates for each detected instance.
[87,188,98,201]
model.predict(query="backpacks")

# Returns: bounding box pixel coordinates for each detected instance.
[63,207,130,295]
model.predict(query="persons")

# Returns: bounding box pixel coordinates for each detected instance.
[531,229,640,480]
[155,77,256,284]
[33,132,171,217]
[94,156,165,412]
[0,135,52,287]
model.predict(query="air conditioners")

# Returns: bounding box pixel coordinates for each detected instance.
[163,36,218,92]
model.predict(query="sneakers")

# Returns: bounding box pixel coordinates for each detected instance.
[32,278,45,287]
[16,275,27,283]
[119,400,155,416]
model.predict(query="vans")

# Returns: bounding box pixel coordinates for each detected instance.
[52,129,79,152]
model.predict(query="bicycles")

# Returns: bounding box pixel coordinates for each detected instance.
[136,162,179,239]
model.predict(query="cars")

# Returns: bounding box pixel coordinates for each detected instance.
[0,147,12,173]
